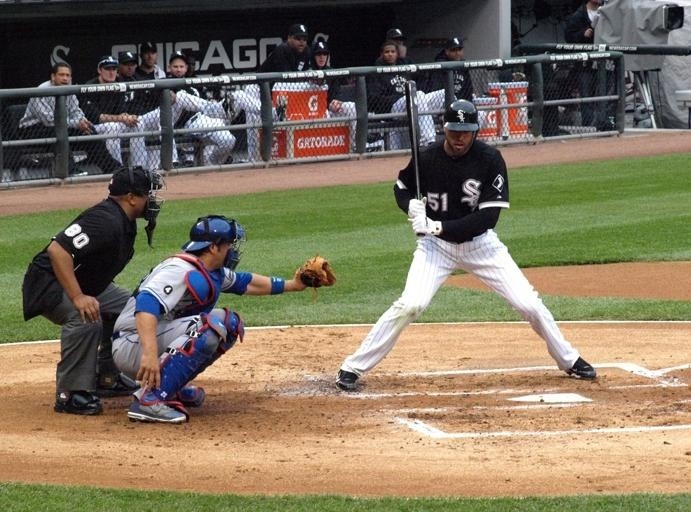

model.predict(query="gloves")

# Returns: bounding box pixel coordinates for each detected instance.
[405,197,442,236]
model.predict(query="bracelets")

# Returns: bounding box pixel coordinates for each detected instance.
[270,276,285,295]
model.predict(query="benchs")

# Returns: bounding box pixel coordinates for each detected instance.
[337,82,441,149]
[3,102,204,170]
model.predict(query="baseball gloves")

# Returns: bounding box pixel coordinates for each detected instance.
[296,257,335,287]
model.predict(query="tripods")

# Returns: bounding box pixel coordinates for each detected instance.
[590,70,659,129]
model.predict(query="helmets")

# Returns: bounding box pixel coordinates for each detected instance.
[181,217,245,270]
[443,99,479,132]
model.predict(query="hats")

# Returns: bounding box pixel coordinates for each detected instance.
[313,41,328,54]
[289,24,308,37]
[169,51,187,63]
[97,56,117,69]
[386,28,402,39]
[118,51,135,63]
[140,42,155,52]
[447,36,463,49]
[107,165,165,220]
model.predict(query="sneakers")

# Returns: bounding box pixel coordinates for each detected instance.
[70,166,88,176]
[335,369,358,391]
[125,398,187,422]
[272,94,287,122]
[216,93,236,120]
[54,390,101,414]
[566,357,595,379]
[95,376,134,395]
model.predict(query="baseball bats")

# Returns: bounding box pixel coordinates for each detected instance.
[406,80,427,236]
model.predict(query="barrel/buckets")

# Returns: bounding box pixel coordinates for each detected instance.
[271,81,329,121]
[471,98,498,138]
[488,81,529,134]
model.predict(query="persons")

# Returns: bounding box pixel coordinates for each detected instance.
[21,165,168,415]
[334,99,596,390]
[110,214,308,424]
